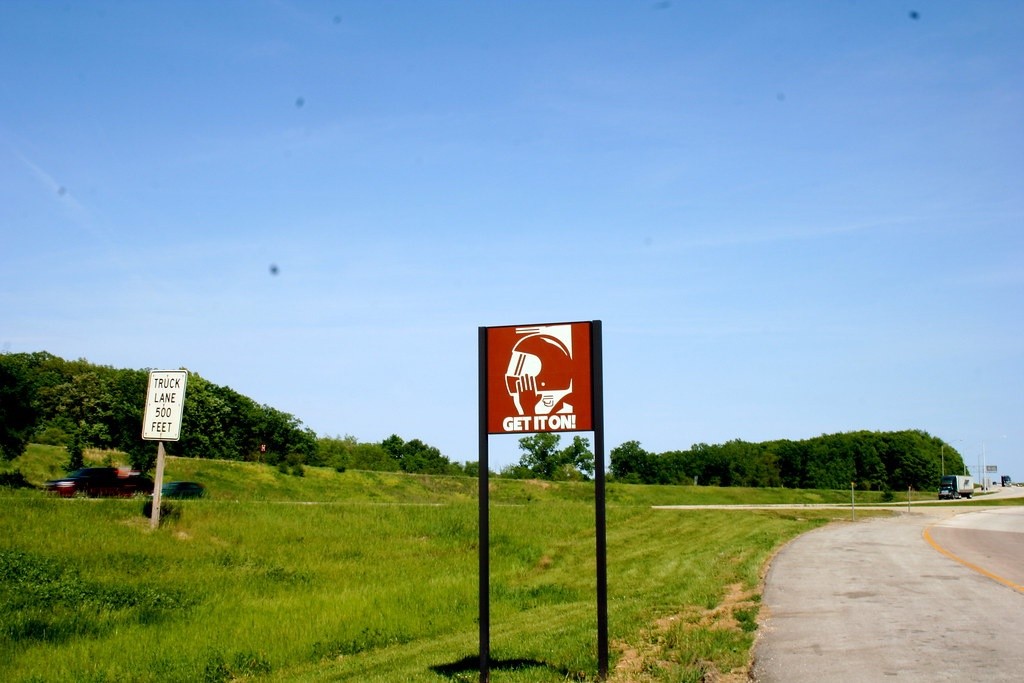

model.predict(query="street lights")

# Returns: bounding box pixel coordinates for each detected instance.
[981,434,1008,493]
[941,438,965,475]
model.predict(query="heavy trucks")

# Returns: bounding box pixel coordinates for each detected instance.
[1001,474,1012,488]
[936,474,976,499]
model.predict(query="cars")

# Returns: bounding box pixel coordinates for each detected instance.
[150,481,204,500]
[44,466,154,502]
